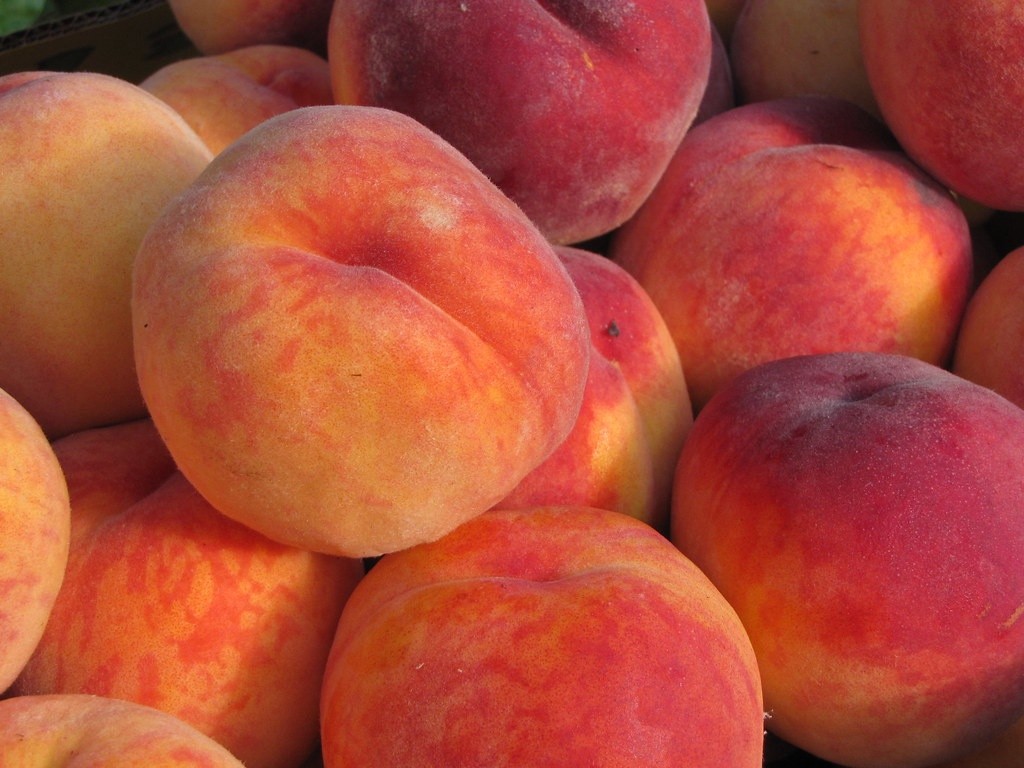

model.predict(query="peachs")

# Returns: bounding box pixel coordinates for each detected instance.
[0,0,1024,768]
